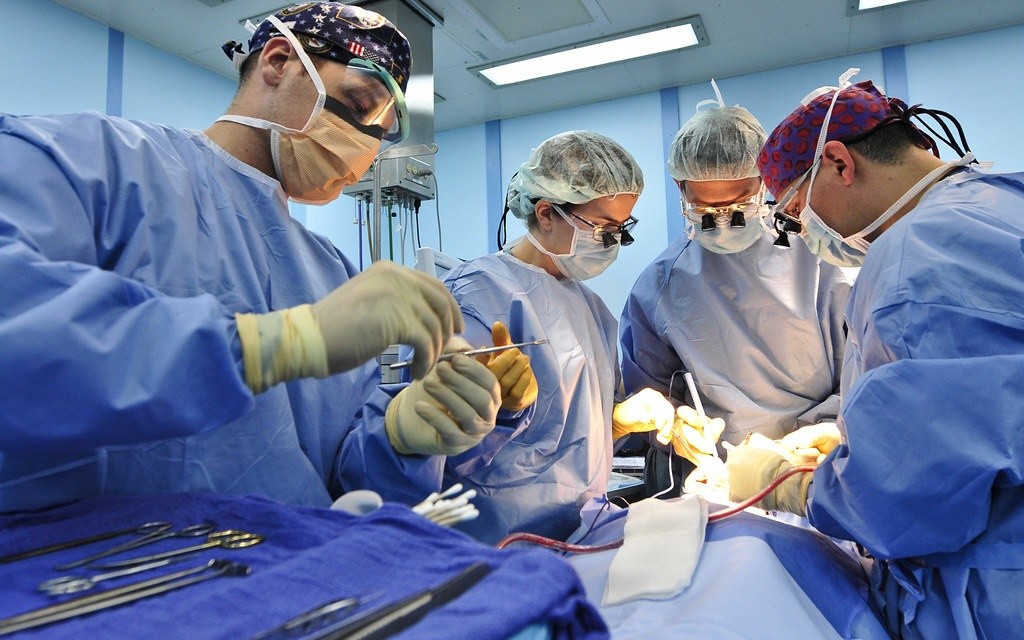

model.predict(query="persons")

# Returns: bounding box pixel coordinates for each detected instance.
[724,70,1023,640]
[615,103,852,459]
[399,129,675,545]
[0,2,499,534]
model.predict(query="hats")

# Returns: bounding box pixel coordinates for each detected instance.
[756,80,940,203]
[248,1,413,99]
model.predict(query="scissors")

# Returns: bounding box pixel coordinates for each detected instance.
[96,529,265,574]
[52,521,216,571]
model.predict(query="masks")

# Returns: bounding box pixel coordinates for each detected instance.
[543,210,622,282]
[684,191,769,255]
[258,50,384,207]
[798,172,877,268]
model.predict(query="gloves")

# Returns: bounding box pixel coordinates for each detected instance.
[473,321,537,410]
[780,422,843,467]
[665,394,726,465]
[233,259,466,396]
[611,388,675,445]
[727,432,813,517]
[384,337,503,456]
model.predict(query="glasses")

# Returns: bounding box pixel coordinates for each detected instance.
[680,185,765,224]
[270,26,411,156]
[559,204,639,243]
[774,137,848,233]
